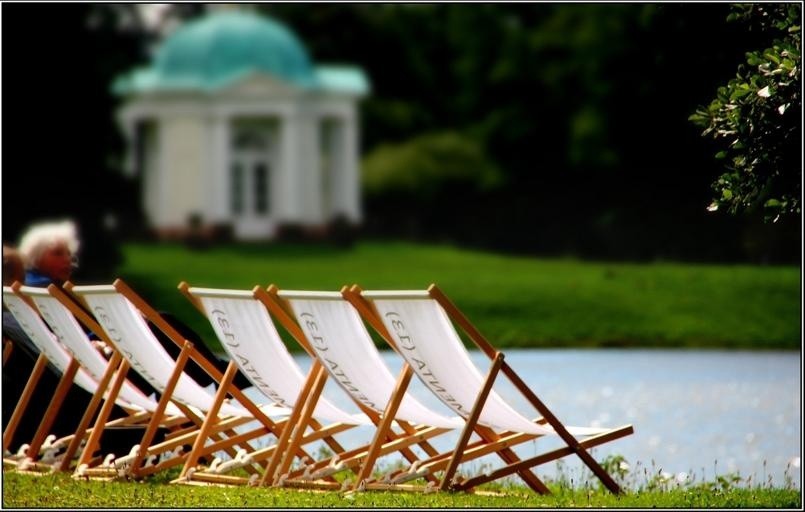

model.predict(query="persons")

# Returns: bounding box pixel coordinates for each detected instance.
[18,219,258,400]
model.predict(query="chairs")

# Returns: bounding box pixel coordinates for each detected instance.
[174,283,441,499]
[63,280,334,486]
[266,281,548,502]
[349,273,635,498]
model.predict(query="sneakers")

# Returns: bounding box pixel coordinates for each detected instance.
[215,359,253,400]
